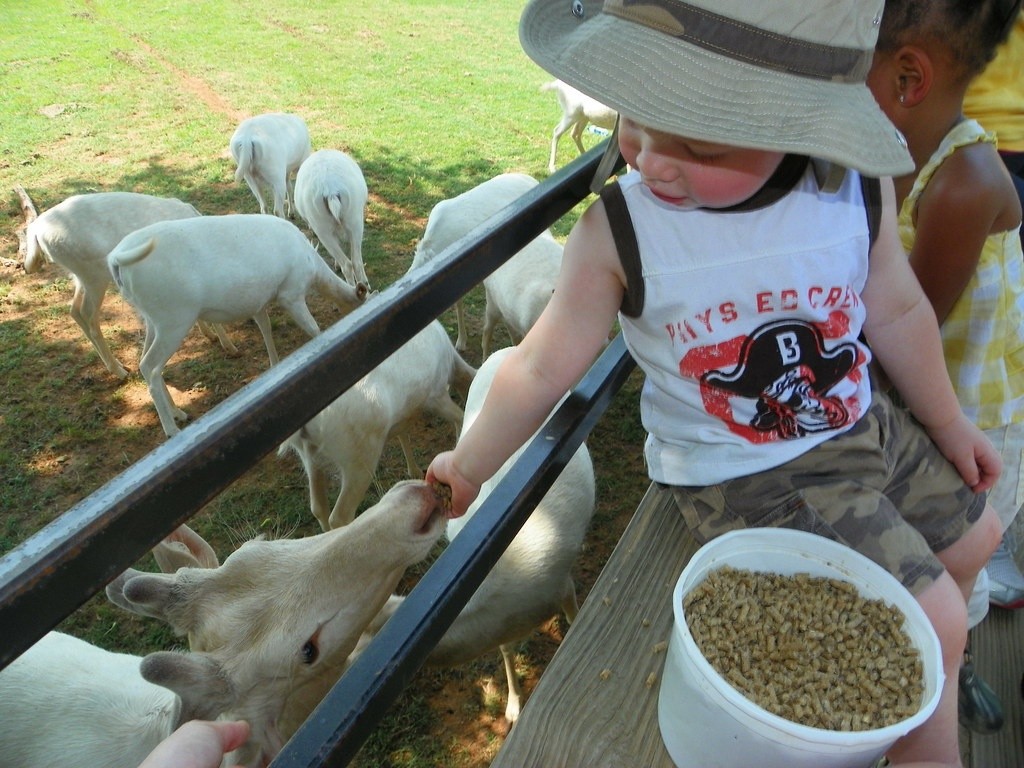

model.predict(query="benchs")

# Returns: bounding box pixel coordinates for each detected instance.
[2,143,1021,767]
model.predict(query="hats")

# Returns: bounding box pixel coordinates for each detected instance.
[518,0,916,177]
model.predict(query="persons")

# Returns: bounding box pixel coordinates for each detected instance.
[422,2,1005,767]
[864,2,1022,616]
[962,1,1022,256]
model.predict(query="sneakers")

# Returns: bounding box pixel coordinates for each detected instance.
[986,533,1024,609]
[958,648,1004,735]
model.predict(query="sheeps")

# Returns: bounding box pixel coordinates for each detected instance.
[151,345,595,723]
[2,478,452,768]
[22,189,241,381]
[108,215,382,449]
[272,285,481,543]
[540,77,619,174]
[392,172,611,371]
[293,148,374,294]
[227,113,310,222]
